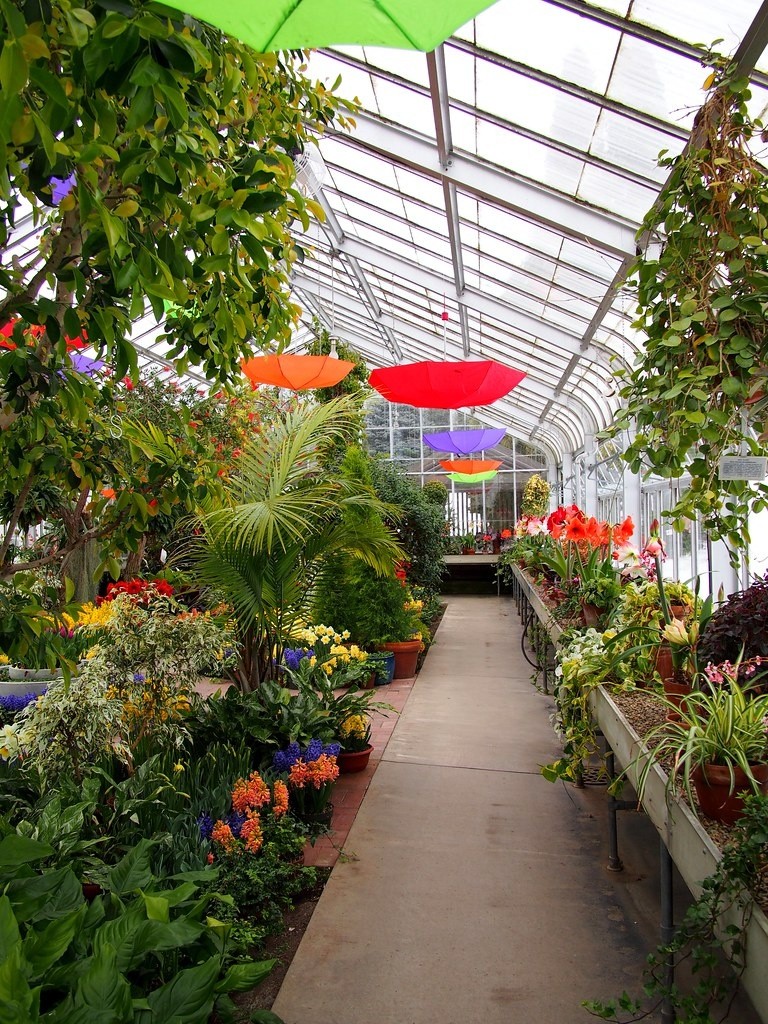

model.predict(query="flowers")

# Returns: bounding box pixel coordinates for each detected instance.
[273,738,340,814]
[231,770,308,862]
[601,519,712,685]
[482,514,551,556]
[547,504,634,589]
[539,540,584,593]
[93,366,321,538]
[393,561,436,654]
[698,656,768,733]
[199,812,273,902]
[0,578,377,751]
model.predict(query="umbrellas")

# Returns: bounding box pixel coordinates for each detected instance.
[421,410,507,455]
[439,452,503,475]
[446,470,499,484]
[369,292,527,409]
[0,318,106,380]
[151,0,499,55]
[21,164,78,205]
[239,332,357,391]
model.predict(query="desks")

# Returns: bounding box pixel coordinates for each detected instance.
[442,554,768,1024]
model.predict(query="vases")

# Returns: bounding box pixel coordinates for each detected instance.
[275,850,305,880]
[649,649,673,680]
[664,713,712,775]
[292,801,334,835]
[664,678,708,714]
[520,559,527,571]
[493,548,501,554]
[9,661,83,680]
[0,665,80,698]
[482,550,488,555]
[558,591,584,619]
[338,743,375,774]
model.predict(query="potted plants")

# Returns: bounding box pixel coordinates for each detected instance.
[462,533,476,555]
[348,557,436,679]
[532,549,561,600]
[348,660,391,690]
[620,577,704,647]
[606,642,768,825]
[368,650,396,685]
[579,576,623,629]
